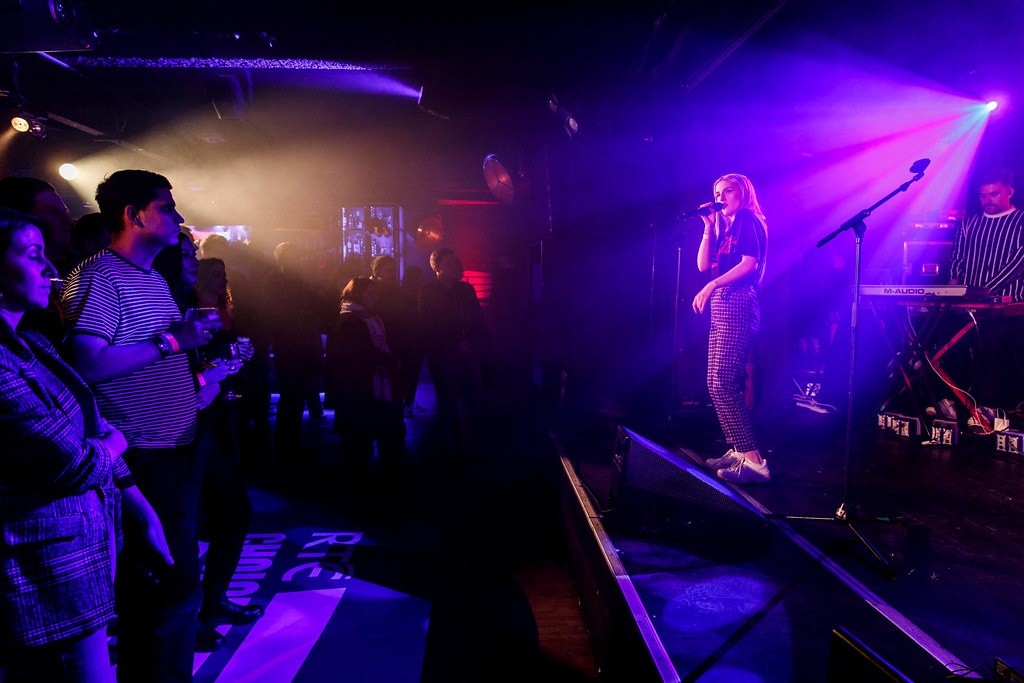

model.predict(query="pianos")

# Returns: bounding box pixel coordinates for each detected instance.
[851,281,1023,435]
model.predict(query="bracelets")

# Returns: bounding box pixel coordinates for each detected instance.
[162,333,180,354]
[151,337,169,359]
[160,335,174,356]
[191,372,201,393]
[197,371,206,388]
[701,233,711,241]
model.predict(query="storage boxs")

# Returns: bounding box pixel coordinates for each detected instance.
[898,241,954,312]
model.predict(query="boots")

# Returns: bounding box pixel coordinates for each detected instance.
[199,529,262,627]
[195,626,216,651]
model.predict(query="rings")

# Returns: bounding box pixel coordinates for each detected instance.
[230,365,235,370]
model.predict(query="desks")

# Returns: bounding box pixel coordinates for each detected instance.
[855,301,1024,436]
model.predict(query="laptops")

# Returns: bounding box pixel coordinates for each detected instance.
[901,241,954,285]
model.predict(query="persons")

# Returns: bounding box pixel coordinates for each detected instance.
[925,166,1024,426]
[691,174,769,483]
[0,169,481,683]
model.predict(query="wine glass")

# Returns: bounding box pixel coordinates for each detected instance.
[223,342,242,401]
[186,307,221,369]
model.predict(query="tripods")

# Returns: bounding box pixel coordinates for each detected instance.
[766,157,932,566]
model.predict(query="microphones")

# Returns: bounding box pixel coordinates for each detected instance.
[678,203,723,221]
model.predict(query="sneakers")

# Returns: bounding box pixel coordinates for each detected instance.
[717,455,770,483]
[926,398,957,418]
[967,406,997,427]
[705,449,745,469]
